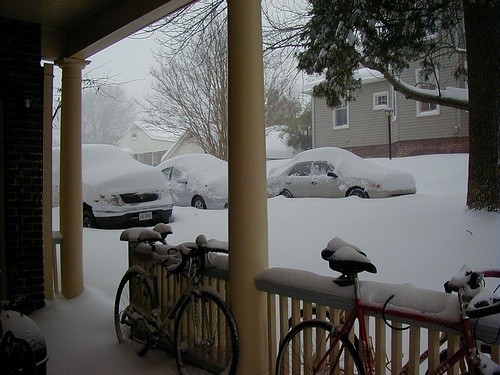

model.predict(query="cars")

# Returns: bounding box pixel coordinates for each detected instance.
[81,144,174,226]
[156,154,229,209]
[275,160,417,199]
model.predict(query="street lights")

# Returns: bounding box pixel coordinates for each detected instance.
[384,106,396,159]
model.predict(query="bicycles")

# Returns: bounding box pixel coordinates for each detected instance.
[272,238,500,375]
[114,232,238,375]
